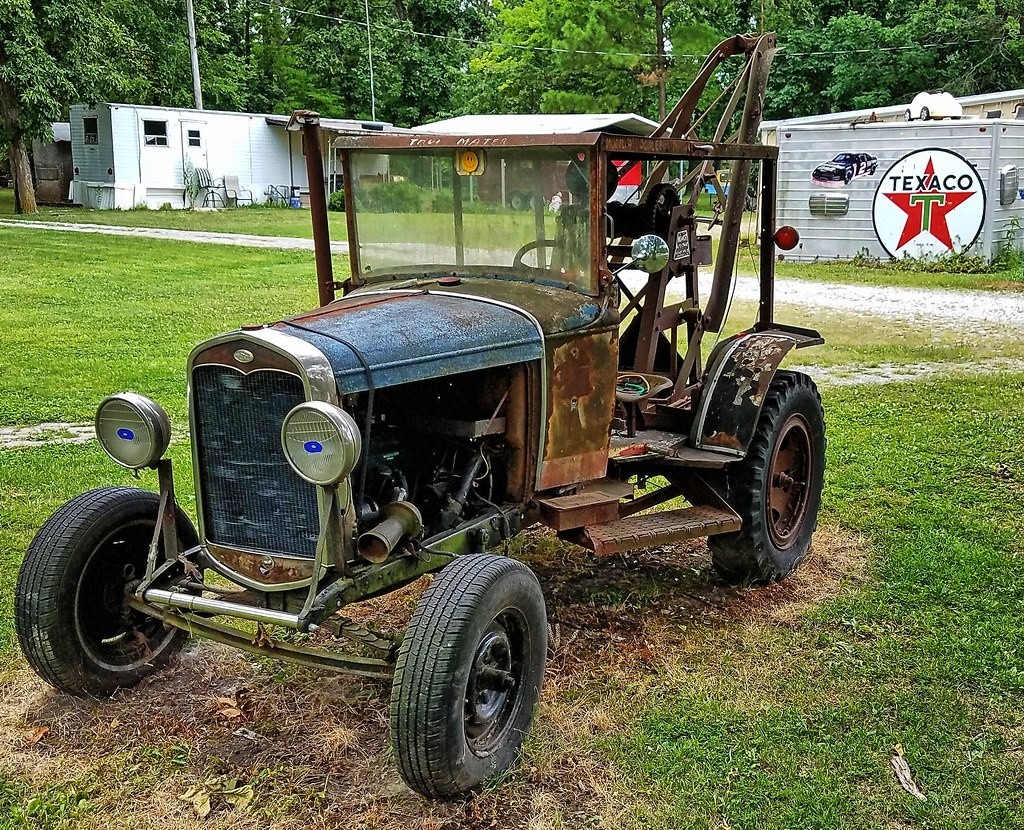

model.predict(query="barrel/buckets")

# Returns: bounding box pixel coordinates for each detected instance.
[292,186,301,198]
[290,197,301,208]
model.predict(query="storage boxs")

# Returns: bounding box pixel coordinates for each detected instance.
[291,198,301,208]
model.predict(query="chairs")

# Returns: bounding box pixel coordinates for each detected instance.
[225,175,253,207]
[196,167,226,208]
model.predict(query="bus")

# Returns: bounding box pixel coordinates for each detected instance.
[477,134,643,216]
